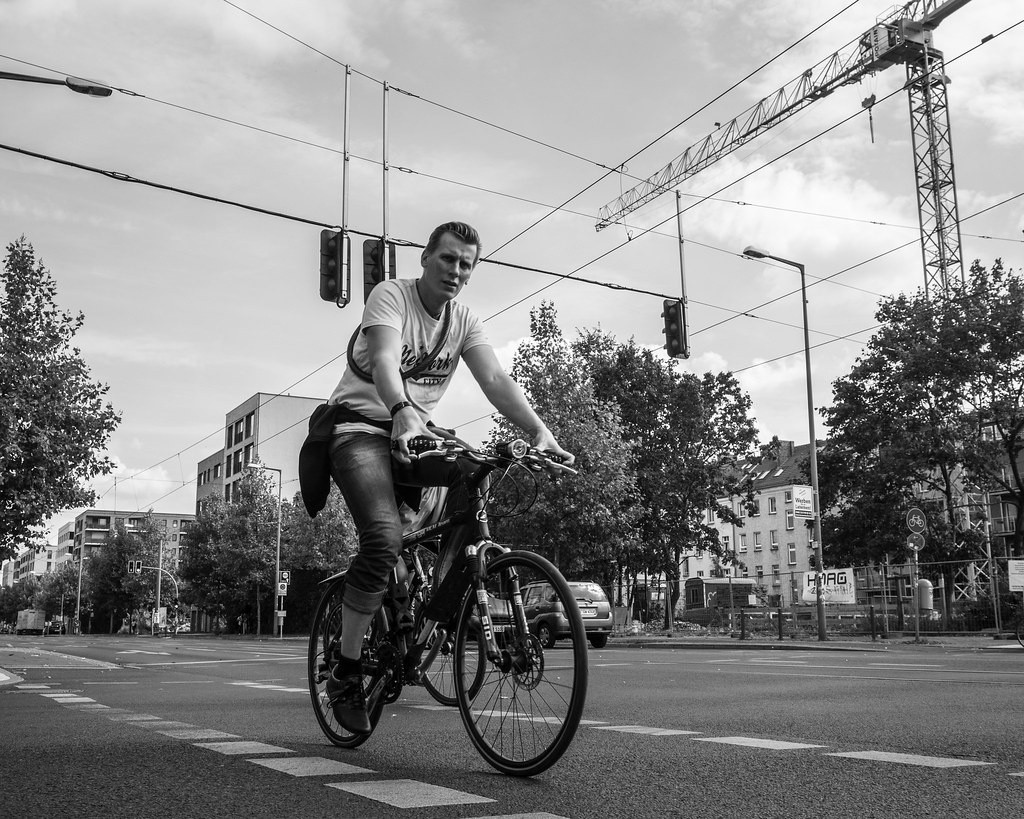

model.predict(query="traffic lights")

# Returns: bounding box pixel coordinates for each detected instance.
[135,560,142,574]
[128,560,134,574]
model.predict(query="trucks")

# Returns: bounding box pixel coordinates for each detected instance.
[16,608,45,635]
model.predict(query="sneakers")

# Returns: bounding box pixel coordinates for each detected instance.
[323,663,372,734]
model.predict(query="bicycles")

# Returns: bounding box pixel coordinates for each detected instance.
[308,436,588,777]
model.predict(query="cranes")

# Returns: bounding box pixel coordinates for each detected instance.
[594,0,1001,621]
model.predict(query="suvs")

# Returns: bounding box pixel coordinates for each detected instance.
[468,596,515,644]
[516,579,616,650]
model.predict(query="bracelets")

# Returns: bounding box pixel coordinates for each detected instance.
[391,402,412,418]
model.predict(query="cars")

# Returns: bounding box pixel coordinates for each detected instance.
[43,621,65,636]
[0,624,16,635]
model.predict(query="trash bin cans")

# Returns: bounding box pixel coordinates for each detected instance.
[916,578,936,611]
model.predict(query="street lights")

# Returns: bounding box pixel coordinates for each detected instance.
[124,523,163,635]
[743,244,830,641]
[247,463,283,637]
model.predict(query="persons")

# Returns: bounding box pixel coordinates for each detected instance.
[312,222,575,734]
